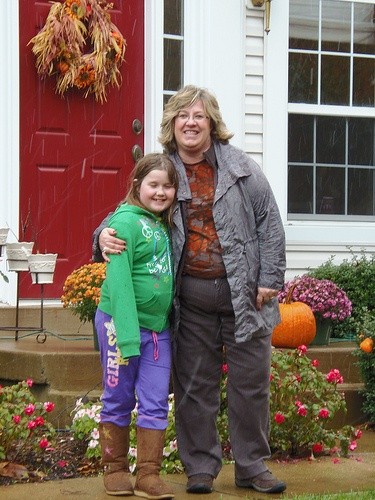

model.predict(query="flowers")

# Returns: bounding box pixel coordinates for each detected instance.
[53,0,123,88]
[277,274,353,322]
[61,261,107,325]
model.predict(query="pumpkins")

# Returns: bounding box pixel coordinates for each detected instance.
[271,284,316,349]
[360,337,374,352]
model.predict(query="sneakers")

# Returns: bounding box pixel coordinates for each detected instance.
[235,470,284,493]
[185,473,214,493]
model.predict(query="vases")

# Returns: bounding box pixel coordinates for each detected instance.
[310,311,334,345]
[91,315,100,351]
[5,242,35,271]
[27,253,58,284]
[0,228,11,257]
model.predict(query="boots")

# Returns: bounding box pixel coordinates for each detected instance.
[134,426,175,499]
[98,421,134,494]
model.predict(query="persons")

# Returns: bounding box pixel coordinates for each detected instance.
[95,154,179,500]
[89,84,286,493]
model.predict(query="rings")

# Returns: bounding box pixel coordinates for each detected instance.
[270,296,274,300]
[103,247,108,252]
